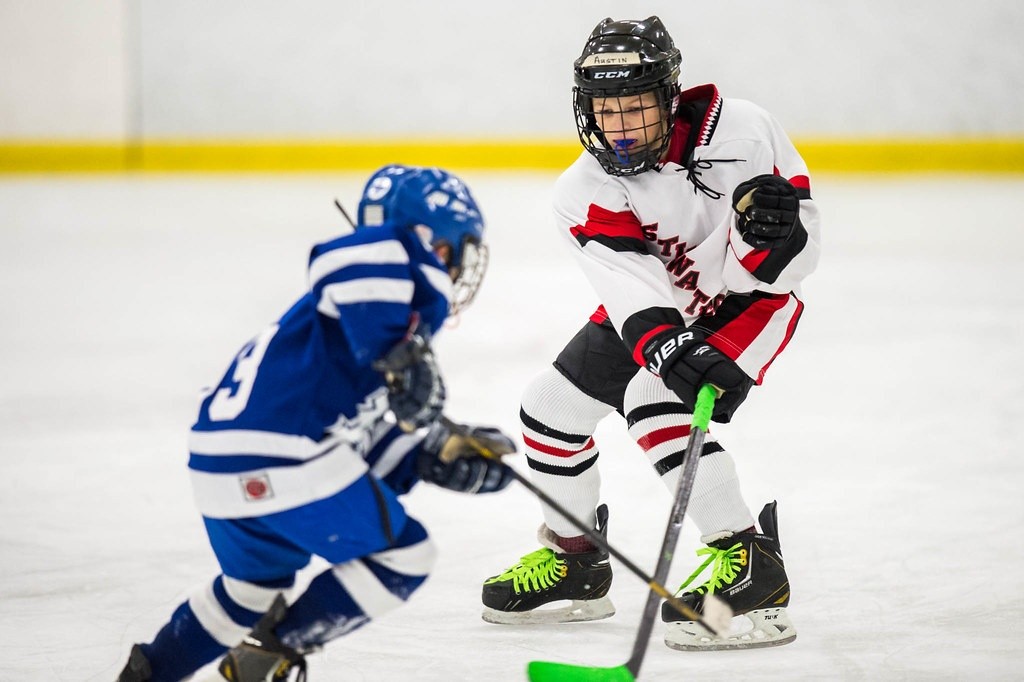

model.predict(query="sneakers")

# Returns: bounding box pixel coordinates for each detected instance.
[117,644,163,682]
[662,500,798,651]
[219,593,307,682]
[481,504,616,625]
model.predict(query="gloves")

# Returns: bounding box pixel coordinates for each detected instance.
[642,327,743,416]
[732,175,800,250]
[385,334,446,432]
[416,425,517,494]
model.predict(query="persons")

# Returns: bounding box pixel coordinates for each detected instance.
[114,165,521,682]
[478,15,823,652]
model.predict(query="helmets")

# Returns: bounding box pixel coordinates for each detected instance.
[572,16,682,177]
[357,165,489,316]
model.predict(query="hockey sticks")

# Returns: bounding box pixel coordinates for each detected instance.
[525,385,717,681]
[437,413,755,638]
